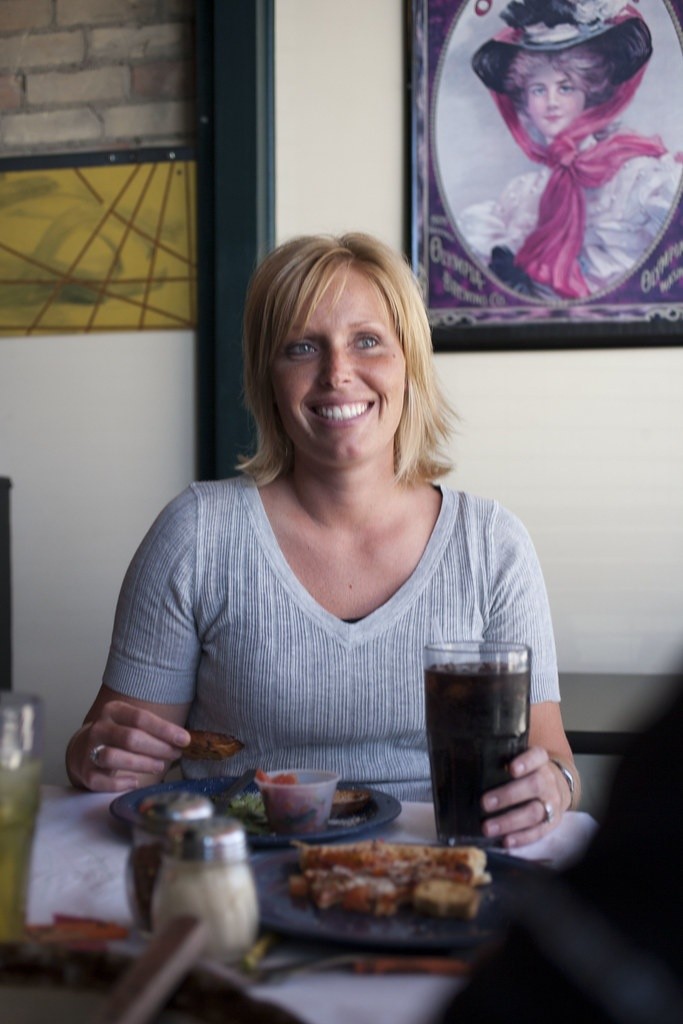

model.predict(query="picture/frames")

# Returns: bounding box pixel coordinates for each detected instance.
[400,0,683,353]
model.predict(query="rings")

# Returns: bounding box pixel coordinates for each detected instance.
[539,799,554,823]
[90,745,105,766]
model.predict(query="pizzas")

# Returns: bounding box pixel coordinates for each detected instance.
[286,841,488,920]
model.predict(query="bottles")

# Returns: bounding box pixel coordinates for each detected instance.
[150,817,260,968]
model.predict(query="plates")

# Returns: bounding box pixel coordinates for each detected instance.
[243,844,544,949]
[109,775,402,850]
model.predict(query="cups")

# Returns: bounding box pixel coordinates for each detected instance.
[423,641,533,848]
[0,693,52,946]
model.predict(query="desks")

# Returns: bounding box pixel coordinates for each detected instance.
[557,672,678,755]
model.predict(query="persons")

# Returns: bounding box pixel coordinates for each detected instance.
[65,233,582,848]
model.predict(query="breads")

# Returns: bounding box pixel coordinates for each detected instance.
[330,790,370,816]
[180,730,244,761]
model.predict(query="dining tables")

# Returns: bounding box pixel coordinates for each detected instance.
[0,783,598,1024]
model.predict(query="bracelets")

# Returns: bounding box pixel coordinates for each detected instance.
[549,758,574,808]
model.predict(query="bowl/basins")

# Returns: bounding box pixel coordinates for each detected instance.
[254,768,341,833]
